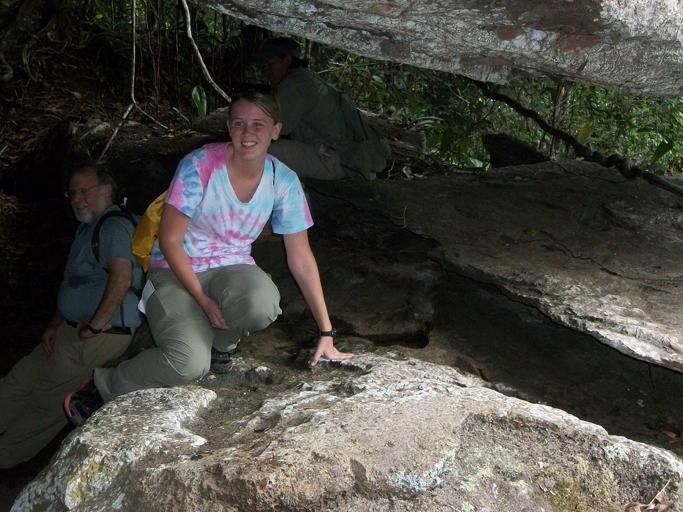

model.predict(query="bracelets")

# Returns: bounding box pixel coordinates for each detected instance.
[86,325,103,334]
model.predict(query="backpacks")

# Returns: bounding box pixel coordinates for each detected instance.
[129,185,169,275]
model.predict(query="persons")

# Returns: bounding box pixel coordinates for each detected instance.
[61,83,355,427]
[0,164,159,475]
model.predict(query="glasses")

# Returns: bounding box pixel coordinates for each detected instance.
[63,182,103,199]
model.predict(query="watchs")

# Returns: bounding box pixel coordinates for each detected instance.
[320,328,336,337]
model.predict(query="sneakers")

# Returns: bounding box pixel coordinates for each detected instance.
[62,378,104,428]
[208,346,233,375]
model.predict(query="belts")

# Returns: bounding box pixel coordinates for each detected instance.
[65,318,133,335]
